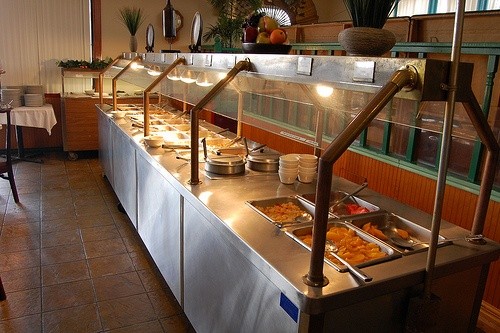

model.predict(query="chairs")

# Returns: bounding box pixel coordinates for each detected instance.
[0,108,19,203]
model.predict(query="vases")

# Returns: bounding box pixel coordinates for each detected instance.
[130,37,138,51]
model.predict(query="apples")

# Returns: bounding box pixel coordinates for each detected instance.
[270,28,288,44]
[257,16,278,34]
[255,31,270,43]
[244,26,257,43]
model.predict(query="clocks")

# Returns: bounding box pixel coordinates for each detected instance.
[166,8,184,44]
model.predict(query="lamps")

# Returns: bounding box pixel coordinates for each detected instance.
[130,62,217,87]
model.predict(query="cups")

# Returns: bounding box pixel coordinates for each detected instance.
[121,52,146,62]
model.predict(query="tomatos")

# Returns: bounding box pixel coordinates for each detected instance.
[345,204,370,215]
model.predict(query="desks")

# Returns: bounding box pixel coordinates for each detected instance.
[0,104,57,163]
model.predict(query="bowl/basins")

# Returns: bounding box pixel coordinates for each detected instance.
[144,136,163,147]
[86,90,95,95]
[111,110,126,118]
[135,90,143,96]
[116,92,127,96]
[278,153,318,184]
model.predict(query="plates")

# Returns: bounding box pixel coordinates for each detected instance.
[1,85,45,107]
[242,42,291,54]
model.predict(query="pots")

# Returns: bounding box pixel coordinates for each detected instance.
[205,151,248,175]
[247,149,280,172]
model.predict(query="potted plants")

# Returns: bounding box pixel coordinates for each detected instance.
[338,0,400,56]
